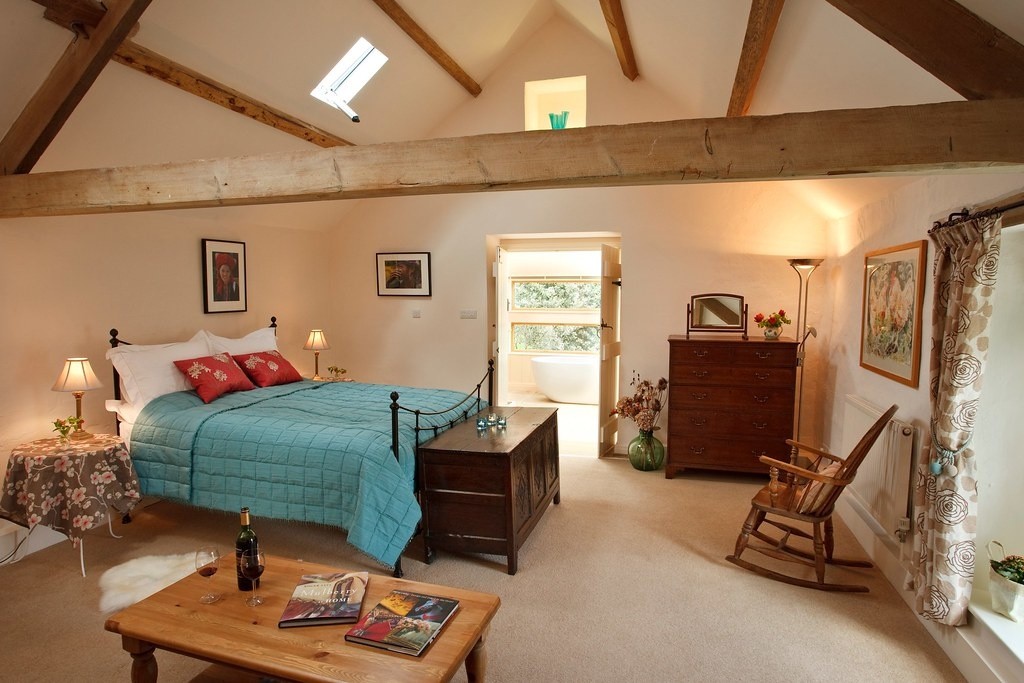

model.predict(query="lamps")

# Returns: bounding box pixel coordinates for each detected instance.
[782,258,826,482]
[303,329,330,381]
[52,357,105,440]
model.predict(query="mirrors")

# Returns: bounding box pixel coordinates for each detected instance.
[686,293,748,341]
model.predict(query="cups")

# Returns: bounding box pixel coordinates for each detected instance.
[497,416,506,429]
[487,412,497,426]
[476,417,486,430]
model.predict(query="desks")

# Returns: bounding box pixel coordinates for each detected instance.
[416,405,561,575]
[104,546,501,683]
[0,434,141,577]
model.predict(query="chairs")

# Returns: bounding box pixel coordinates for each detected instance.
[725,404,900,592]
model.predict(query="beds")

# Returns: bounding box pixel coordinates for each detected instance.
[109,317,496,578]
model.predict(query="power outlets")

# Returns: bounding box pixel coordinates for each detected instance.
[412,310,420,318]
[460,310,477,319]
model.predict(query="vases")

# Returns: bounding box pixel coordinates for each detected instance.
[763,327,783,340]
[56,438,70,452]
[628,429,664,472]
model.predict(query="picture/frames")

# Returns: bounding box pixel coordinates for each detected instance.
[859,239,929,388]
[201,237,248,314]
[375,252,431,296]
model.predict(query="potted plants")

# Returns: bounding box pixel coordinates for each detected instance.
[329,366,347,381]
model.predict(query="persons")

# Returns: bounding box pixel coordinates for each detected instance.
[385,260,420,290]
[212,253,239,301]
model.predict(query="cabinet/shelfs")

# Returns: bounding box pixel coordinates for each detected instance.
[665,334,800,482]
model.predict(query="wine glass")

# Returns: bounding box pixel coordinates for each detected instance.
[240,548,265,606]
[195,545,222,604]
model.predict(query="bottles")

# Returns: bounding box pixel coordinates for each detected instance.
[235,507,260,591]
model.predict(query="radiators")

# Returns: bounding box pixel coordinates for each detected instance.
[840,395,915,544]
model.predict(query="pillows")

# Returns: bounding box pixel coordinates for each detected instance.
[105,329,212,414]
[797,461,843,514]
[207,326,277,357]
[172,352,256,404]
[231,350,304,387]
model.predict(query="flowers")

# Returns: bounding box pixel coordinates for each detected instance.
[754,311,791,328]
[608,372,667,432]
[51,416,85,437]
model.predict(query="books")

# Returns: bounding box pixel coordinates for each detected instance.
[345,589,459,657]
[279,572,370,627]
